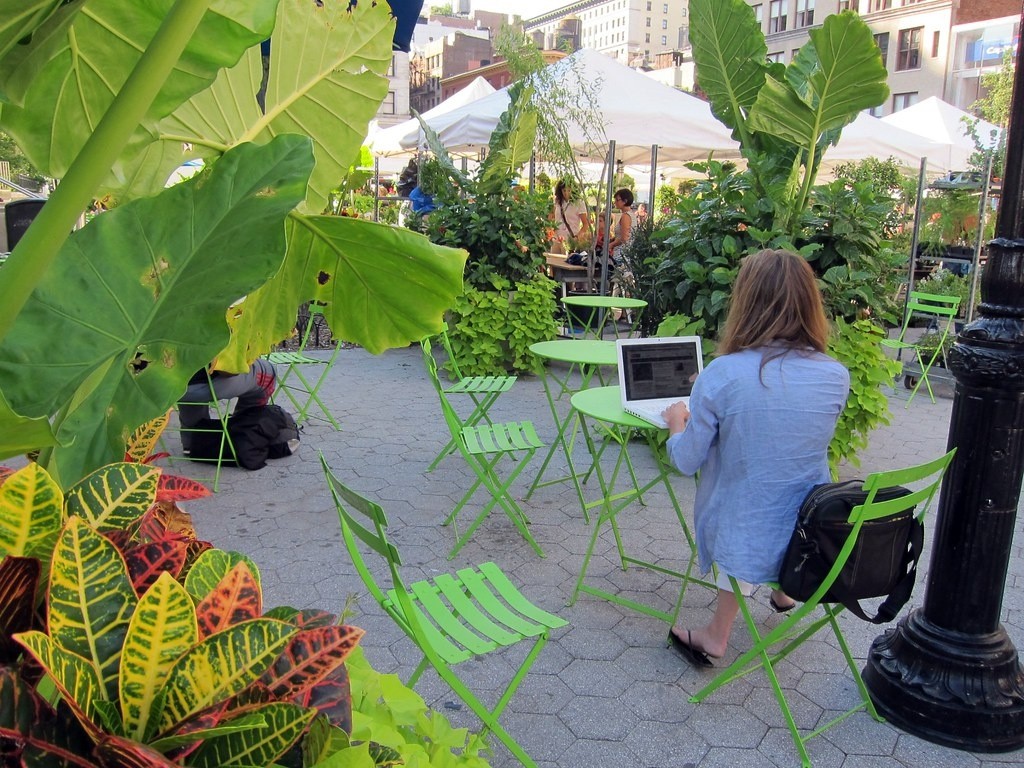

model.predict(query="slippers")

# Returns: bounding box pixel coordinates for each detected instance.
[669,626,715,668]
[769,591,795,613]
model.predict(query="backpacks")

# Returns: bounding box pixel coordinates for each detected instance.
[397,157,418,197]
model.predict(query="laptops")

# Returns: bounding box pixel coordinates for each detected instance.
[617,336,704,429]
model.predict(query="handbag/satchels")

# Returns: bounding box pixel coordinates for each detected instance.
[188,405,300,467]
[777,479,924,626]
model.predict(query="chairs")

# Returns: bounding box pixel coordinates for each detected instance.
[686,445,958,768]
[878,290,962,410]
[317,447,572,768]
[418,338,549,562]
[157,363,240,493]
[417,321,519,474]
[256,304,343,433]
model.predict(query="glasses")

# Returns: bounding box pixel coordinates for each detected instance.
[615,198,622,202]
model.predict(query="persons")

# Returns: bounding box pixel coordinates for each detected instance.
[175,360,277,455]
[601,188,638,324]
[658,247,852,670]
[546,178,589,241]
[635,204,649,225]
[588,211,616,256]
[610,161,635,191]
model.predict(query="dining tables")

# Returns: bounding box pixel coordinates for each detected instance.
[556,296,649,402]
[522,339,647,524]
[563,386,722,648]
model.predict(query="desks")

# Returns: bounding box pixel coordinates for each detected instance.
[546,255,599,297]
